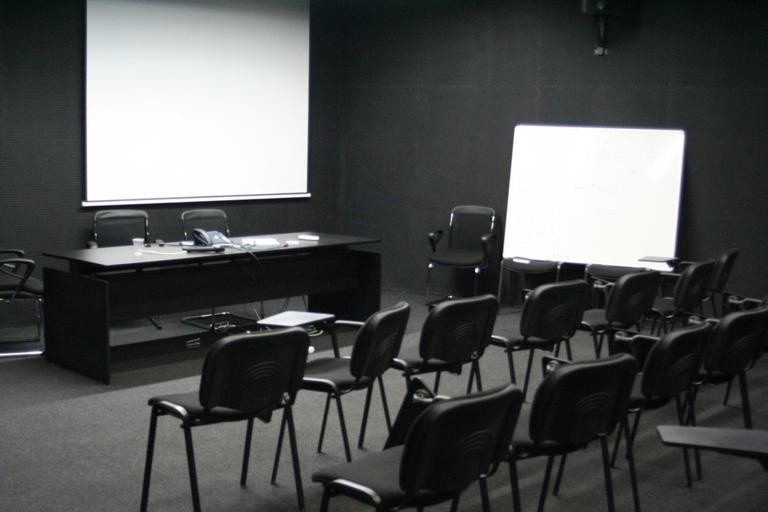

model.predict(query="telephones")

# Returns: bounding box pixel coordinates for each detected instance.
[192,228,233,249]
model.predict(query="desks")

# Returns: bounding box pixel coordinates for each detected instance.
[42,229,382,384]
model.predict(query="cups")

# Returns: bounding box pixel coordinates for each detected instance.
[133,238,144,256]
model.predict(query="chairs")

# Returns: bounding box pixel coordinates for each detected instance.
[139,326,310,512]
[310,383,524,512]
[181,208,230,240]
[355,294,499,448]
[424,204,495,297]
[607,322,712,487]
[87,209,150,248]
[554,270,661,358]
[464,280,589,396]
[269,301,411,484]
[0,248,46,358]
[682,309,768,480]
[650,262,715,334]
[699,250,740,315]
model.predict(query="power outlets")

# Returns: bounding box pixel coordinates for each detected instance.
[503,354,637,512]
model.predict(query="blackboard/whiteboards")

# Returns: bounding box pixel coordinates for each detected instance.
[502,123,684,273]
